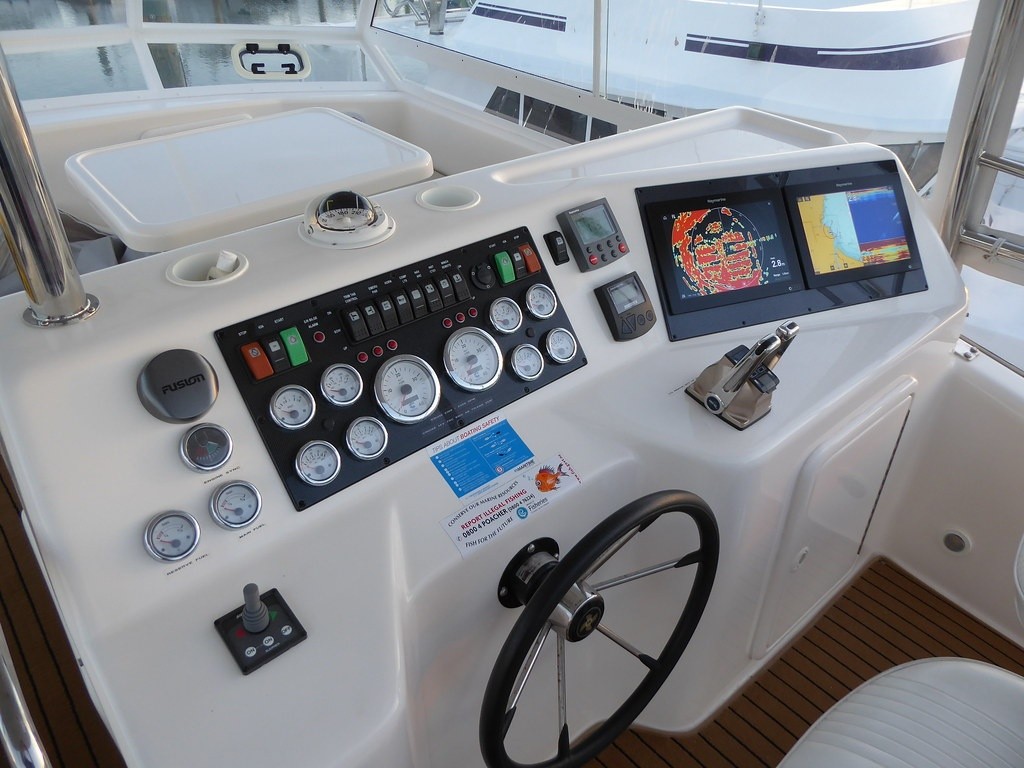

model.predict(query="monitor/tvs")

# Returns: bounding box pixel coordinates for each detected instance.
[646,187,805,314]
[782,172,923,289]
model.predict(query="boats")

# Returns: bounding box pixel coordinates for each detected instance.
[347,0,1024,214]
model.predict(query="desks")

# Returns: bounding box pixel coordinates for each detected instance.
[64,107,434,253]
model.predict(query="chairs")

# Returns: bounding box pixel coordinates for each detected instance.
[777,657,1024,768]
